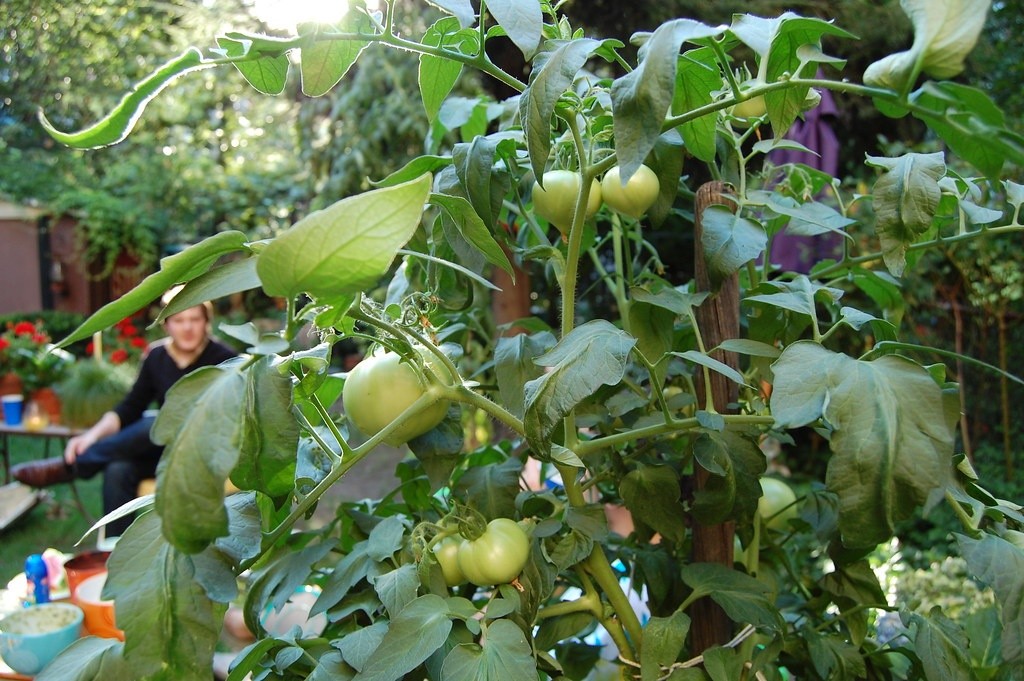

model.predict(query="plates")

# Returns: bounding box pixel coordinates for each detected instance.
[7,573,70,600]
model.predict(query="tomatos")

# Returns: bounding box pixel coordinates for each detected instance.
[531,160,660,235]
[753,475,799,530]
[342,344,454,447]
[726,87,768,129]
[432,516,528,587]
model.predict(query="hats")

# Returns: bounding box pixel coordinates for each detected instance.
[161,284,214,320]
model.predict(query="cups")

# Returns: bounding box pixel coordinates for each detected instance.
[1,394,24,424]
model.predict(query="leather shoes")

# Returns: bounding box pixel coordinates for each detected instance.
[11,457,76,487]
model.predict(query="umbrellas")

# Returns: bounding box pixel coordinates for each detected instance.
[757,62,840,277]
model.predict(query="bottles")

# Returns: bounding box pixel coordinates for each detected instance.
[24,554,50,605]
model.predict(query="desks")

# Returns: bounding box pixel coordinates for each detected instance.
[0,421,98,526]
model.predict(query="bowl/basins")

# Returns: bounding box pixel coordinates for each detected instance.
[75,571,126,644]
[63,552,112,600]
[0,603,84,676]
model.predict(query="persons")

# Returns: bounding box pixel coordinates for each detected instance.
[472,425,663,665]
[11,284,239,537]
